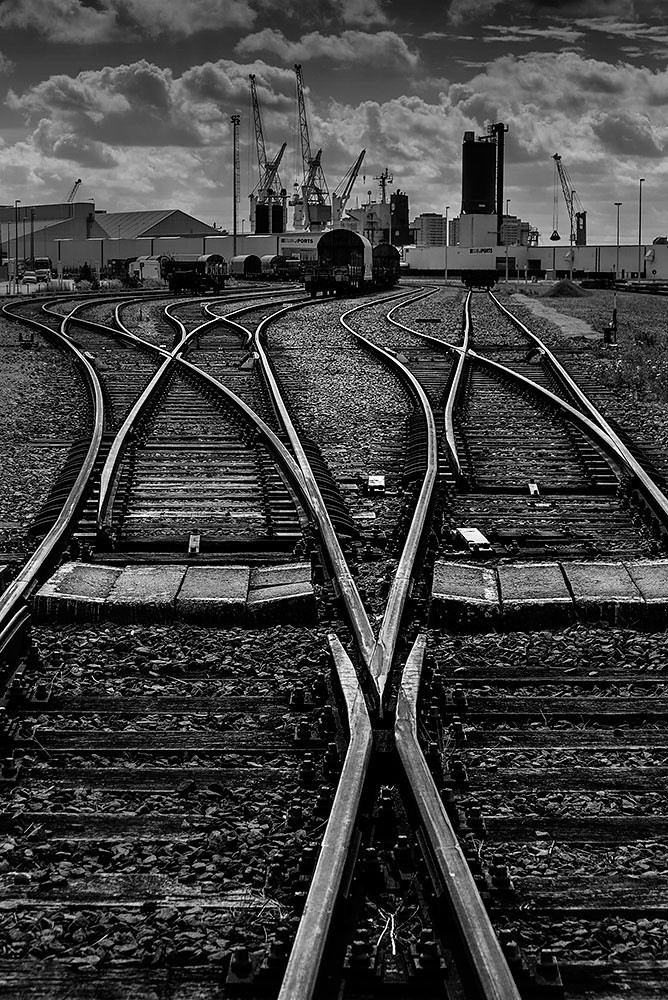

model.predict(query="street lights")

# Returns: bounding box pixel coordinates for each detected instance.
[637,178,646,283]
[506,199,511,216]
[569,190,576,280]
[446,207,450,217]
[7,220,12,259]
[14,199,21,284]
[614,202,622,275]
[23,206,37,271]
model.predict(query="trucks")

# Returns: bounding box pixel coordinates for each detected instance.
[7,258,28,279]
[34,256,53,282]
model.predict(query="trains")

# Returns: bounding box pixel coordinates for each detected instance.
[101,228,403,298]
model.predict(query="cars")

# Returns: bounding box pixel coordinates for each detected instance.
[23,271,37,285]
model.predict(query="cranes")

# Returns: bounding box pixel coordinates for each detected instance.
[550,152,589,246]
[62,178,82,202]
[247,62,368,231]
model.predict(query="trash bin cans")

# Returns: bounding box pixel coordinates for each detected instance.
[546,269,553,280]
